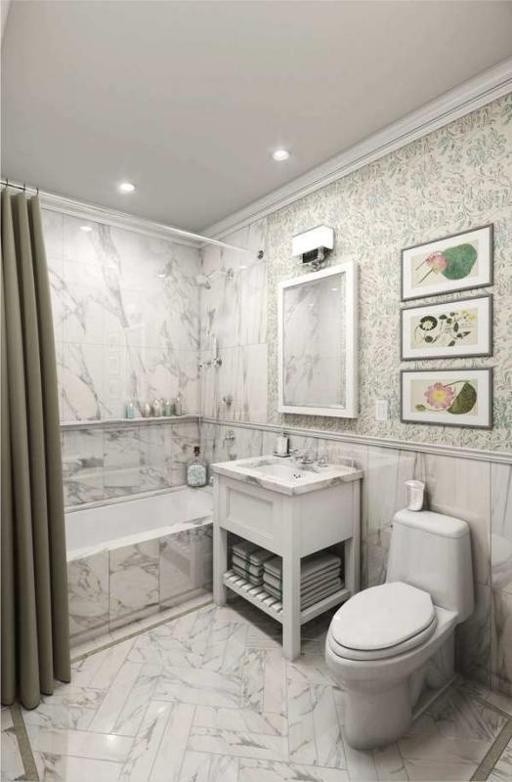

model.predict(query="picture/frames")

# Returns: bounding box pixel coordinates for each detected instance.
[396,221,498,304]
[396,365,497,433]
[395,292,496,362]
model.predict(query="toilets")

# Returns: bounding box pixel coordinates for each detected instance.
[322,507,479,752]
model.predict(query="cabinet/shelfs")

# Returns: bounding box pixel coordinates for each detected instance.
[210,474,362,663]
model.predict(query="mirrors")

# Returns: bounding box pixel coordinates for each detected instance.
[273,257,365,422]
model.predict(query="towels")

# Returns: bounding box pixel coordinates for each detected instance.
[229,538,343,609]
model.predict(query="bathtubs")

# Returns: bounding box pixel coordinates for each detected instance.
[65,485,215,566]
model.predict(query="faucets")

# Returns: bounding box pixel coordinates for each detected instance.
[288,448,315,465]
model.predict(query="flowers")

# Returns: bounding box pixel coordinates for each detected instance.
[413,240,480,285]
[411,307,477,347]
[413,377,480,416]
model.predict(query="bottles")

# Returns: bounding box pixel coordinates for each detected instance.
[125,391,187,420]
[274,430,289,455]
[187,445,207,487]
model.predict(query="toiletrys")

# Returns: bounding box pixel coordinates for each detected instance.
[276,431,289,454]
[124,391,188,419]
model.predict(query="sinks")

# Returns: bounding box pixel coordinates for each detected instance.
[236,457,323,480]
